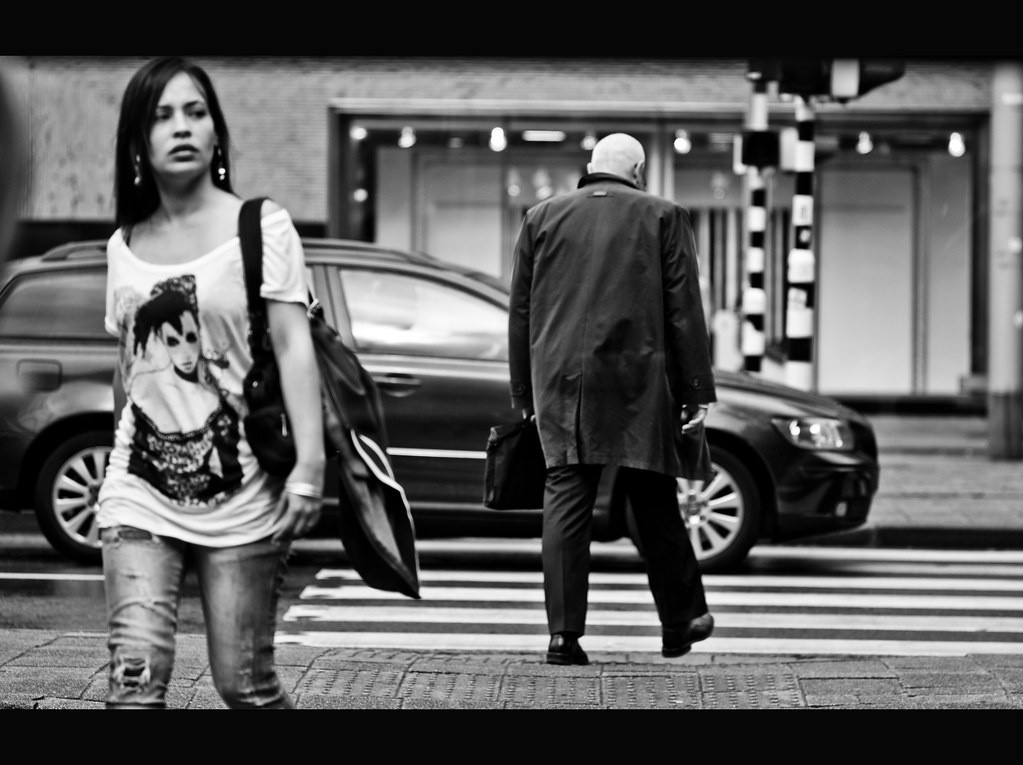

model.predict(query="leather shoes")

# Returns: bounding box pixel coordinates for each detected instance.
[661,613,714,658]
[546,634,589,665]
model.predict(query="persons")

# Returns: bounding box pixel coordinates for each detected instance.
[508,133,718,667]
[93,59,333,708]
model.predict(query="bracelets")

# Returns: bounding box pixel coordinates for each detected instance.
[698,405,711,409]
[284,481,324,501]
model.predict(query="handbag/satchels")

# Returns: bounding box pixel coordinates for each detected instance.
[238,192,323,482]
[482,413,545,511]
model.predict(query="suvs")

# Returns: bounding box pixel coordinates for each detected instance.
[0,240,880,577]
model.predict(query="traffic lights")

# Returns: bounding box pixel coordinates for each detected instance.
[827,59,907,106]
[744,132,780,167]
